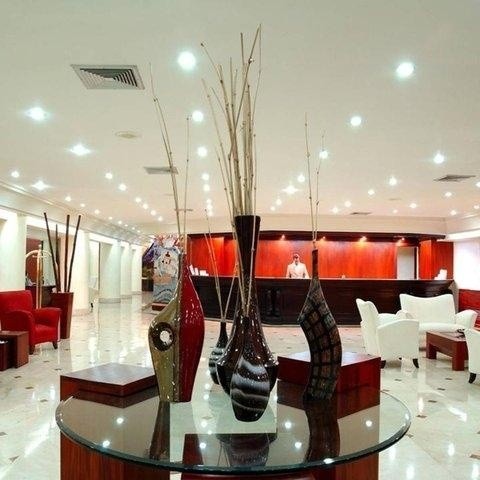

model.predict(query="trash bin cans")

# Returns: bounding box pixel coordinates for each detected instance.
[1,342,9,370]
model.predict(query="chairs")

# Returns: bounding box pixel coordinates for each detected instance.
[0,289,63,354]
[354,294,480,383]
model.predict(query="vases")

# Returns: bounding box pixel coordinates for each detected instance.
[50,291,74,339]
[148,253,205,402]
[208,214,279,422]
[143,214,357,422]
[297,250,342,421]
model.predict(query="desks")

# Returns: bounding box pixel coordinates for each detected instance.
[56,345,410,478]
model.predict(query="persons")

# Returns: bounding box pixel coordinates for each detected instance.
[285,254,312,280]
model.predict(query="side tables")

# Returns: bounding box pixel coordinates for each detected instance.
[1,330,30,368]
